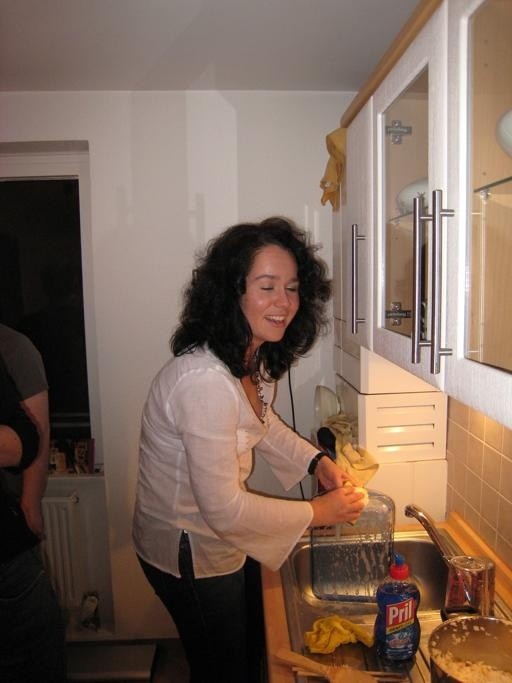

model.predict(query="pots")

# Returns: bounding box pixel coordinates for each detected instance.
[427,604,512,683]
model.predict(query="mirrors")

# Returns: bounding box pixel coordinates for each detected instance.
[379,60,428,346]
[465,3,512,373]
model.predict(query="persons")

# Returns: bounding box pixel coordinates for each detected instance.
[1,320,55,539]
[128,214,367,683]
[1,384,68,681]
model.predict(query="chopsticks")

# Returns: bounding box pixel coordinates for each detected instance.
[291,666,405,682]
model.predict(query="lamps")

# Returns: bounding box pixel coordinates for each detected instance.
[496,109,512,158]
[396,179,429,214]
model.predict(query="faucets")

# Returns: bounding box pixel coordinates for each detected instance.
[404,503,483,612]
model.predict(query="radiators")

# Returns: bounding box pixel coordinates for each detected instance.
[40,488,87,602]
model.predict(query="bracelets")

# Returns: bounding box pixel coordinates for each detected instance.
[308,451,326,476]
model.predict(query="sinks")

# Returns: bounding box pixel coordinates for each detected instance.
[280,529,493,613]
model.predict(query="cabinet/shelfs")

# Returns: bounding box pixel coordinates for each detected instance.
[331,93,449,528]
[372,2,512,433]
